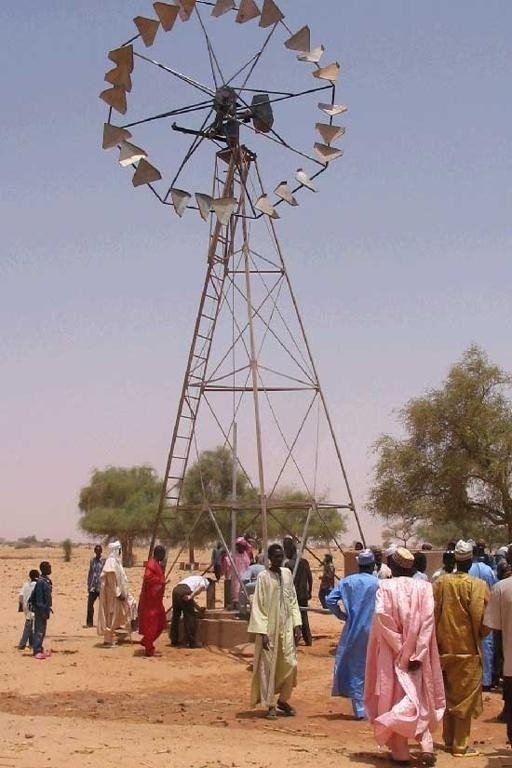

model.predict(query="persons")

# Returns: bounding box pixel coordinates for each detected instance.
[138,545,166,657]
[465,542,492,690]
[369,544,392,578]
[97,539,134,648]
[413,554,428,584]
[285,540,313,646]
[319,553,335,610]
[247,545,304,721]
[170,576,214,648]
[482,543,512,745]
[496,559,508,579]
[427,544,456,580]
[365,547,446,768]
[16,570,38,649]
[324,550,379,720]
[28,560,54,659]
[211,539,223,582]
[432,540,485,759]
[497,545,508,557]
[227,538,249,609]
[82,544,106,630]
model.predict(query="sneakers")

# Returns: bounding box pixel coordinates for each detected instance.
[42,651,49,656]
[35,653,44,658]
[267,707,278,719]
[453,747,479,756]
[445,744,454,751]
[279,701,293,714]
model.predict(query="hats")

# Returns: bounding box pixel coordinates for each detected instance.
[493,547,508,555]
[356,549,373,563]
[454,541,471,559]
[392,547,413,568]
